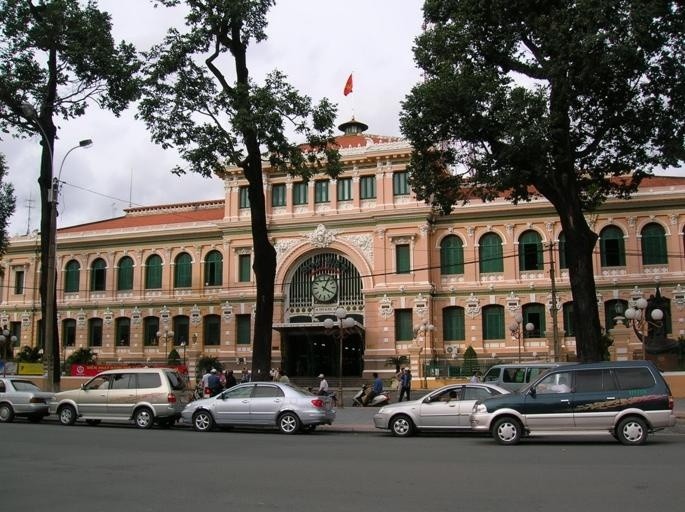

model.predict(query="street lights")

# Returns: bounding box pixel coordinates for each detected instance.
[324,307,357,407]
[510,313,535,365]
[0,329,17,377]
[415,318,439,389]
[16,99,94,396]
[180,337,187,366]
[625,297,665,359]
[155,330,174,361]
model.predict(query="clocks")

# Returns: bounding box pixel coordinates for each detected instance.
[311,274,337,302]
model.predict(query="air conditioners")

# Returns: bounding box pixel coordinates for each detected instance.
[444,344,458,354]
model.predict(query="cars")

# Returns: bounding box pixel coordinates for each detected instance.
[179,380,337,434]
[372,383,514,439]
[0,376,57,423]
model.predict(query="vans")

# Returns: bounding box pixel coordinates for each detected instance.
[481,362,583,395]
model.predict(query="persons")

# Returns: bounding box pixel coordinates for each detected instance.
[398,367,411,401]
[363,372,382,407]
[195,366,291,400]
[471,371,481,383]
[315,373,328,396]
[394,367,408,399]
[440,391,459,401]
[538,376,571,394]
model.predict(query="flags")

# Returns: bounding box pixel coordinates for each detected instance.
[343,73,353,96]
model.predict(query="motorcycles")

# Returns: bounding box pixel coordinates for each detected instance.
[352,383,390,407]
[305,385,339,408]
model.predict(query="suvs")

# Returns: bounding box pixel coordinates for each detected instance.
[469,359,677,446]
[46,367,193,431]
